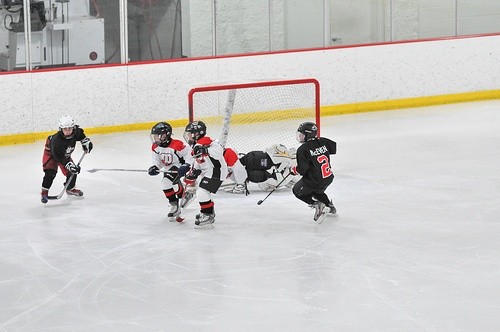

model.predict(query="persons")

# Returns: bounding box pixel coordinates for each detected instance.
[41,115,93,207]
[183,120,228,229]
[289,122,337,223]
[217,140,302,196]
[148,122,196,221]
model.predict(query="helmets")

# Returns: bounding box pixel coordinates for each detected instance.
[183,121,206,146]
[297,122,318,143]
[150,123,172,147]
[59,114,76,140]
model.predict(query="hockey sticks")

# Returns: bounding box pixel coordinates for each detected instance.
[257,173,291,205]
[169,156,196,222]
[47,150,87,199]
[87,169,179,173]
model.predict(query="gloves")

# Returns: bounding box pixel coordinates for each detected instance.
[65,161,80,175]
[178,163,190,176]
[289,166,298,175]
[187,168,202,179]
[148,165,160,176]
[80,137,94,154]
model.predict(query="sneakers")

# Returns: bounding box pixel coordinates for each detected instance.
[167,199,182,221]
[194,214,214,230]
[182,192,197,208]
[309,201,331,224]
[328,199,336,216]
[195,208,216,220]
[41,187,49,207]
[64,182,84,199]
